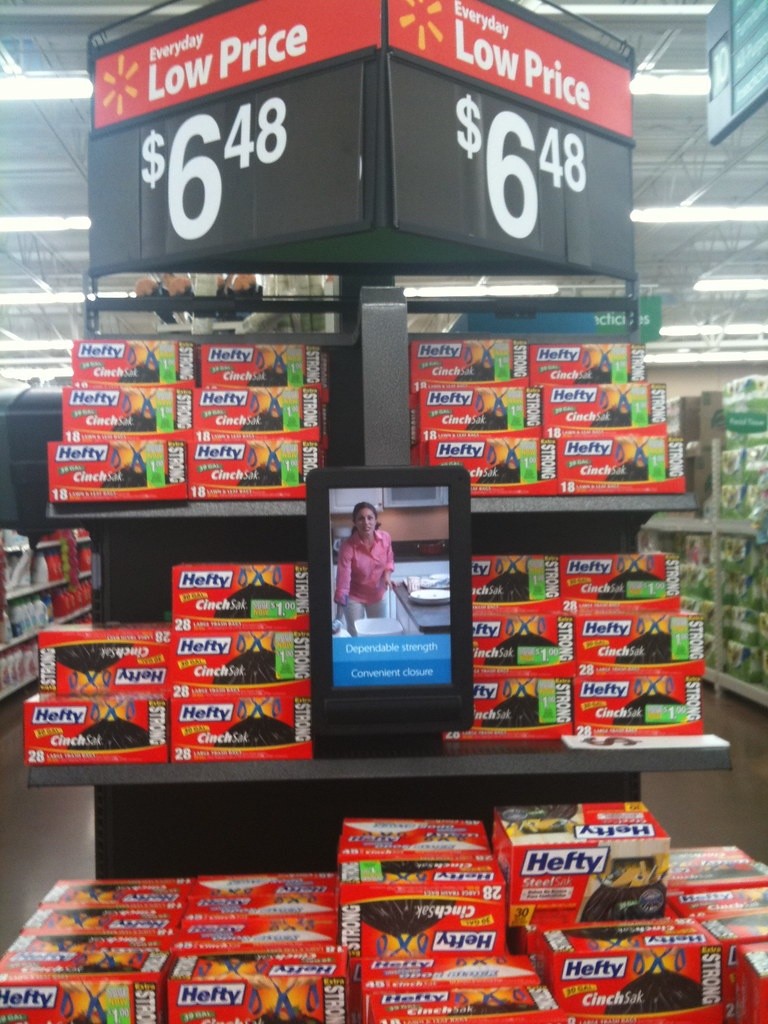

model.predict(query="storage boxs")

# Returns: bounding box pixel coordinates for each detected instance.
[24,563,314,765]
[445,551,705,740]
[409,340,686,496]
[0,804,768,1024]
[47,340,328,503]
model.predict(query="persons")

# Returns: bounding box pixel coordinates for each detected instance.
[332,503,395,637]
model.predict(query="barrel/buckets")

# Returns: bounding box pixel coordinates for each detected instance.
[354,618,403,637]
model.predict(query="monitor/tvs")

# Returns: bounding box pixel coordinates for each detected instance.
[307,466,474,739]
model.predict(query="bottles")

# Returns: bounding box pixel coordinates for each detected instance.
[47,544,93,625]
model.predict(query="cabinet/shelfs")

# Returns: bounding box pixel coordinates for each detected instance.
[0,0,768,1024]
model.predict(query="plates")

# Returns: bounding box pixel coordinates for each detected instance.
[430,574,449,579]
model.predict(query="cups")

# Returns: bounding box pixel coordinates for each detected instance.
[408,576,420,594]
